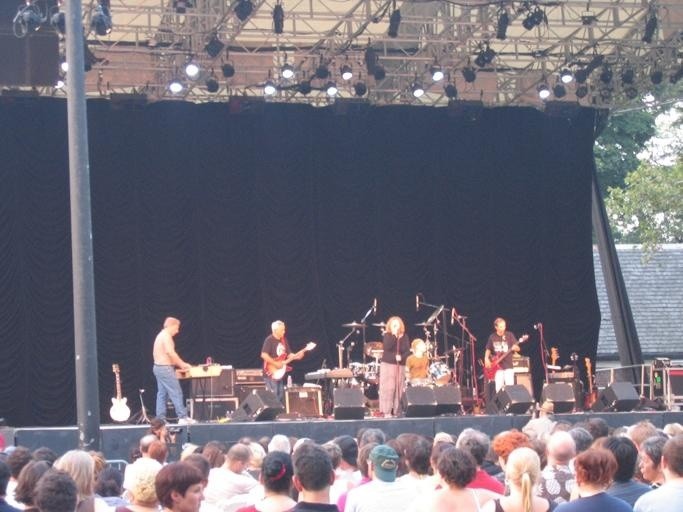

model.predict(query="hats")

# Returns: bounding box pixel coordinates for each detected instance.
[538,401,554,414]
[368,444,400,482]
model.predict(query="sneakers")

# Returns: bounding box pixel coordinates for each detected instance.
[177,417,196,425]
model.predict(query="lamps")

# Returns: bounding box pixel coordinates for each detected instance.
[353,83,366,97]
[222,48,235,77]
[206,66,218,92]
[444,84,457,98]
[561,68,573,85]
[169,77,182,92]
[172,1,194,15]
[429,67,444,81]
[474,49,494,68]
[272,4,283,35]
[324,80,337,94]
[340,65,353,80]
[389,9,403,37]
[496,14,508,40]
[522,11,543,30]
[411,83,424,96]
[234,2,253,21]
[281,65,294,79]
[642,19,657,43]
[575,56,681,99]
[316,64,330,79]
[537,85,549,99]
[365,46,376,76]
[462,71,475,82]
[185,59,197,76]
[554,84,564,97]
[264,82,275,94]
[207,33,225,57]
[300,80,310,94]
[375,66,384,80]
[12,2,113,74]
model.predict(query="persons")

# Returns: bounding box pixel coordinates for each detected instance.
[203,355,213,364]
[261,320,305,401]
[483,318,520,413]
[152,317,197,425]
[405,338,429,385]
[1,416,683,510]
[379,316,411,418]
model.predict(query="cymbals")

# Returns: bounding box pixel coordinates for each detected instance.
[371,324,388,326]
[415,323,433,327]
[341,324,367,329]
[444,348,465,352]
[362,342,384,357]
[428,356,450,358]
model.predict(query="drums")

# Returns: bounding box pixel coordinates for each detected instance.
[350,363,366,379]
[428,361,452,385]
[408,379,433,386]
[366,361,379,384]
[363,381,379,404]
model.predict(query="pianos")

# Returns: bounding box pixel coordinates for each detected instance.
[304,369,351,380]
[175,365,222,378]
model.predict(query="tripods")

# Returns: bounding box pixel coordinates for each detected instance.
[463,336,487,415]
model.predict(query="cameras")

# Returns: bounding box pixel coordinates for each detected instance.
[165,426,183,434]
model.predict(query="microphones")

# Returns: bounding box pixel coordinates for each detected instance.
[373,298,377,317]
[534,322,541,329]
[416,296,419,313]
[451,307,455,326]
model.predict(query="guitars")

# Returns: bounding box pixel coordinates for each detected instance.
[264,340,317,382]
[110,364,130,421]
[583,357,597,410]
[484,334,529,382]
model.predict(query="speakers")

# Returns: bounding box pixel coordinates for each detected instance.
[432,383,462,416]
[401,385,437,417]
[590,382,641,413]
[231,388,283,421]
[486,384,533,415]
[539,382,576,414]
[333,388,365,420]
